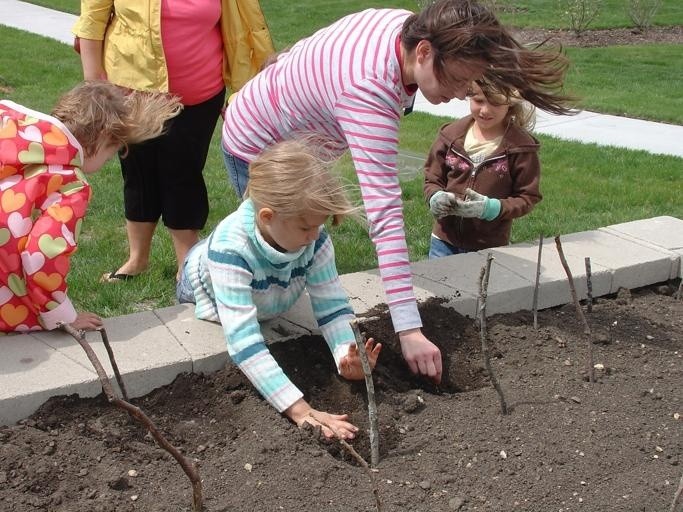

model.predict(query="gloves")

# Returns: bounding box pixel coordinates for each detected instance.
[429,188,502,222]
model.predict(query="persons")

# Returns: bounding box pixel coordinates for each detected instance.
[219,1,587,387]
[70,0,277,286]
[0,76,185,335]
[177,130,382,443]
[422,76,543,261]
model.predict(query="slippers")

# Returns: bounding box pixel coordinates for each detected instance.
[99,267,138,285]
